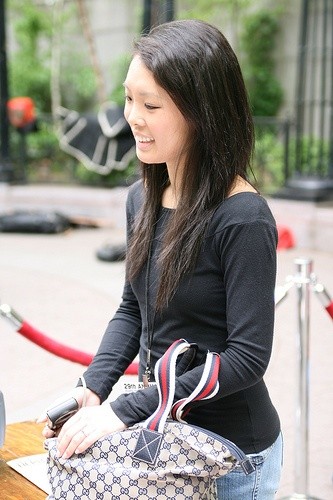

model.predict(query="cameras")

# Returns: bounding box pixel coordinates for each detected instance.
[46,397,79,437]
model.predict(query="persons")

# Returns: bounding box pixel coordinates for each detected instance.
[37,20,284,500]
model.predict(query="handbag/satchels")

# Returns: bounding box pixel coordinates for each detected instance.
[44,339,254,500]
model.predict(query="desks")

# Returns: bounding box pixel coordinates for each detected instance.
[0,420,47,500]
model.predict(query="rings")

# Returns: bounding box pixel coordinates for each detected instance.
[79,428,88,440]
